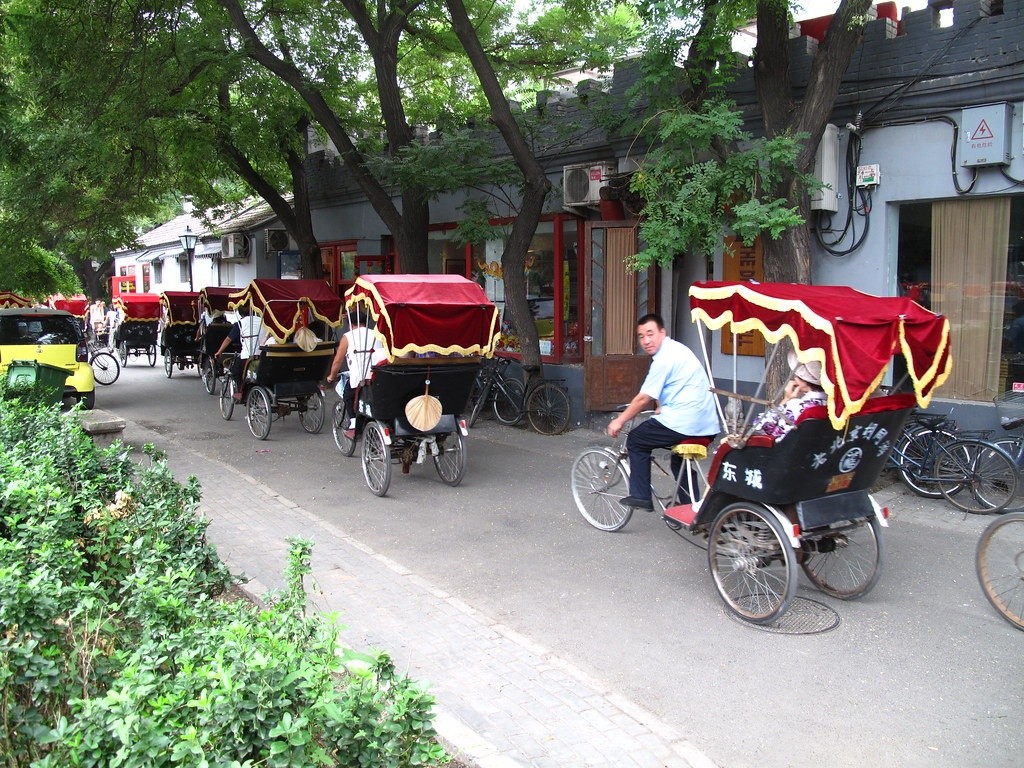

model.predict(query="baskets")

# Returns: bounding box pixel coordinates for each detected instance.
[481,359,506,379]
[993,389,1024,430]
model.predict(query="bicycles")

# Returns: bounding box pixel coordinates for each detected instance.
[880,384,1024,514]
[976,512,1024,635]
[468,352,570,435]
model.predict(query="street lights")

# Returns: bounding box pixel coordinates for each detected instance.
[179,225,199,292]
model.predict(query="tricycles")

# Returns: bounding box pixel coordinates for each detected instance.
[0,292,120,385]
[571,276,956,621]
[109,293,159,368]
[159,279,344,440]
[332,273,501,498]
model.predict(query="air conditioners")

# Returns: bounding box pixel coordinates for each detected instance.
[563,161,616,206]
[221,233,244,259]
[264,228,300,252]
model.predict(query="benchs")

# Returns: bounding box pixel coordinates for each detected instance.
[200,323,234,355]
[114,321,158,341]
[707,393,916,503]
[161,324,199,348]
[243,340,336,388]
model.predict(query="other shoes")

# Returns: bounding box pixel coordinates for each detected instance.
[234,393,241,399]
[619,496,654,511]
[344,430,355,439]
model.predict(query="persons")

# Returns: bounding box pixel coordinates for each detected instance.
[692,345,828,513]
[327,311,387,440]
[28,298,120,353]
[194,304,214,343]
[214,305,268,399]
[1002,301,1024,403]
[607,313,721,512]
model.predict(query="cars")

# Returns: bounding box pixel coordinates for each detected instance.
[0,309,95,410]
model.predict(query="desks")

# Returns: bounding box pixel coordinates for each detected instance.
[357,357,482,420]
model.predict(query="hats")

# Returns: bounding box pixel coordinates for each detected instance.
[787,348,822,385]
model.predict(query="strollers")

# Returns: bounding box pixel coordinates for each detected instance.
[93,322,120,351]
